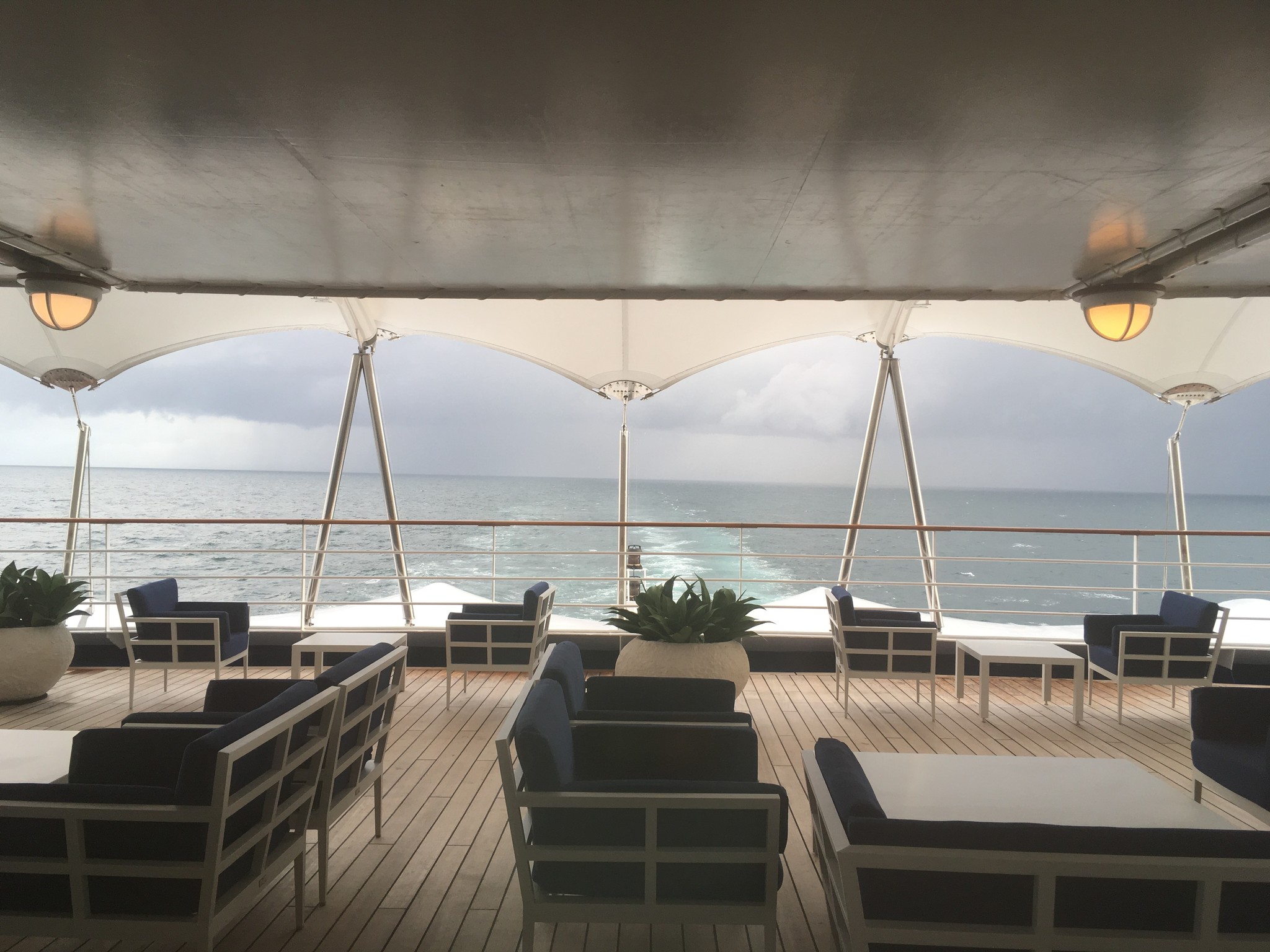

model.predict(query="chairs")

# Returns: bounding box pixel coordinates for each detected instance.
[529,642,755,730]
[1082,590,1230,724]
[119,642,410,905]
[113,578,251,711]
[444,581,558,711]
[0,680,340,951]
[1185,686,1270,829]
[824,585,937,722]
[494,679,791,951]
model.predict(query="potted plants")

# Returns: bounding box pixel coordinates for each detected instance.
[0,558,96,705]
[598,571,777,699]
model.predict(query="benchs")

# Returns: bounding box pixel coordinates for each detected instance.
[804,739,1270,952]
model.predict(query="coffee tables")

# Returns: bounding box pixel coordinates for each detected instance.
[0,727,84,785]
[953,638,1086,724]
[290,631,408,695]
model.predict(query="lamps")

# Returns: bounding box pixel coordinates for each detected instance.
[14,266,112,333]
[1069,279,1165,346]
